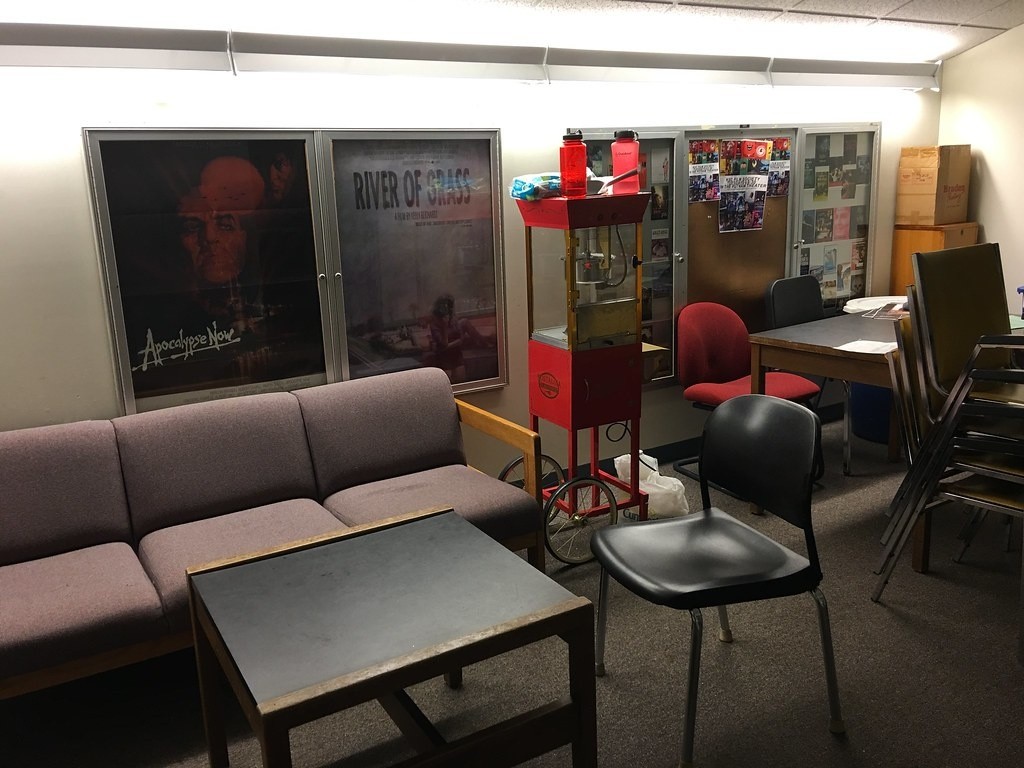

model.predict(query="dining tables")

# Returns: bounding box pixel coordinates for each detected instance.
[765,274,852,476]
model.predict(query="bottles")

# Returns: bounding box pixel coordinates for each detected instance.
[559,127,587,197]
[610,129,640,195]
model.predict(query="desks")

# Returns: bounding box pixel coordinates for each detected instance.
[185,506,595,768]
[746,309,1024,463]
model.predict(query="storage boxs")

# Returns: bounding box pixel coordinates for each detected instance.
[893,144,971,225]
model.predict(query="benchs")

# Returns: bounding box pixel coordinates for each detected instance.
[0,366,546,700]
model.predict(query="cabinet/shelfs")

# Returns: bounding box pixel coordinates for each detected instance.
[887,221,979,295]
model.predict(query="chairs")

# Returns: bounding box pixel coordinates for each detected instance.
[671,302,824,500]
[869,243,1024,604]
[591,394,846,768]
